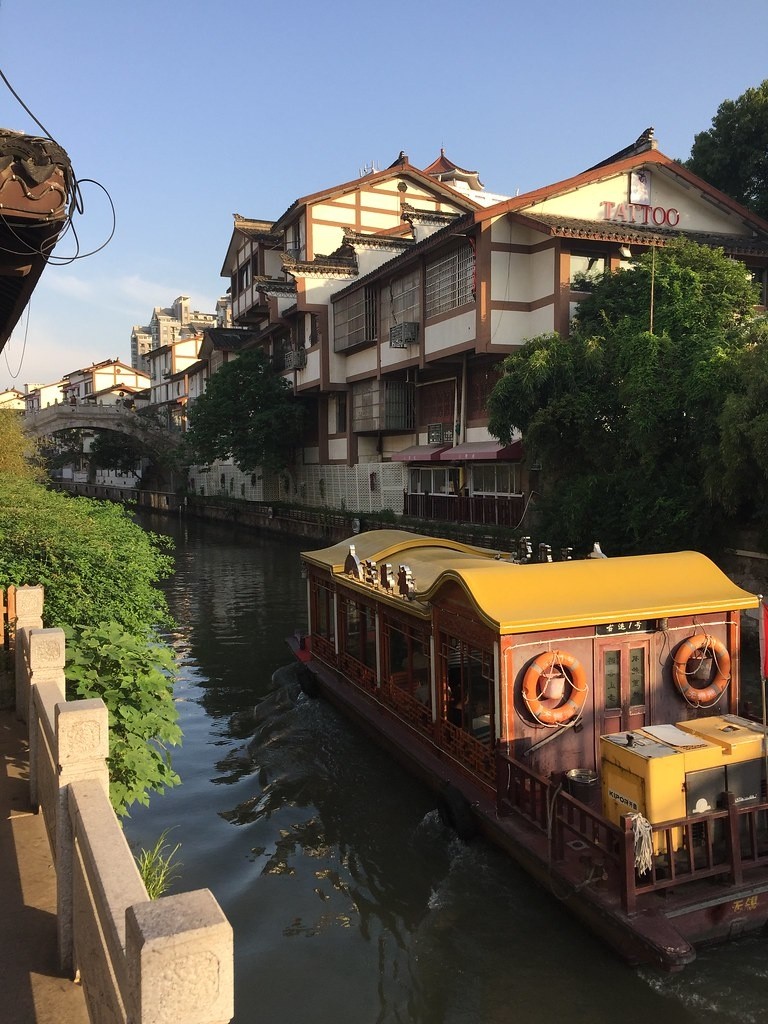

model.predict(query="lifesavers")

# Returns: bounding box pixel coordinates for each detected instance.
[521,649,587,725]
[671,633,731,704]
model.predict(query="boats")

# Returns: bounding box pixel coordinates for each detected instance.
[282,528,768,974]
